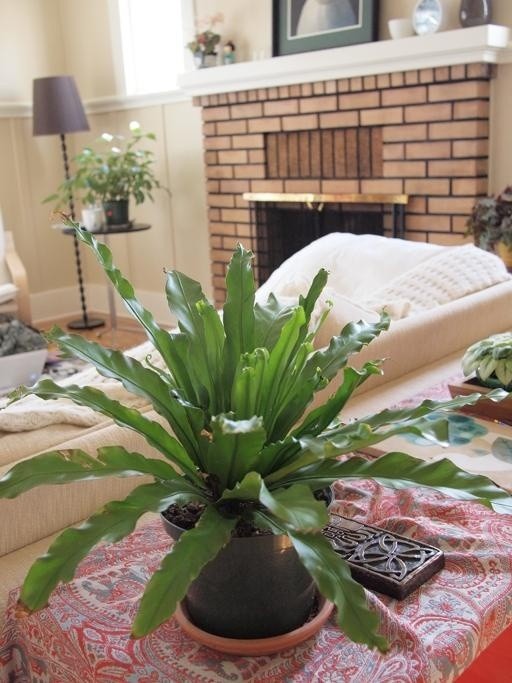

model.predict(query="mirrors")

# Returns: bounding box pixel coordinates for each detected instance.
[412,0,442,36]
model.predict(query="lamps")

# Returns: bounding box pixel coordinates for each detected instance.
[32,75,104,330]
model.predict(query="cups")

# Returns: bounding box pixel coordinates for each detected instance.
[388,19,415,38]
[82,208,103,232]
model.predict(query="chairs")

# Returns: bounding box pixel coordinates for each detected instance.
[0,211,32,328]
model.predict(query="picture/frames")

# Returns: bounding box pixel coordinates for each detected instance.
[271,1,379,58]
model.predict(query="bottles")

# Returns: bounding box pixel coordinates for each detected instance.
[410,1,493,37]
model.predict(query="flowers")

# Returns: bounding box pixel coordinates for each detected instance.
[186,31,220,53]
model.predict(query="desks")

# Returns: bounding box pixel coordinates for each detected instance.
[0,324,512,682]
[63,222,151,338]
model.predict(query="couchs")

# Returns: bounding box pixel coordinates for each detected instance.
[0,232,512,563]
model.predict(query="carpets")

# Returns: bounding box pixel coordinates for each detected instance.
[41,349,90,382]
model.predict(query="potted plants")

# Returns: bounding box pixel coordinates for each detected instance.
[40,120,172,226]
[0,241,512,656]
[463,186,512,267]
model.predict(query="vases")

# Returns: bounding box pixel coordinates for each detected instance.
[194,52,217,69]
